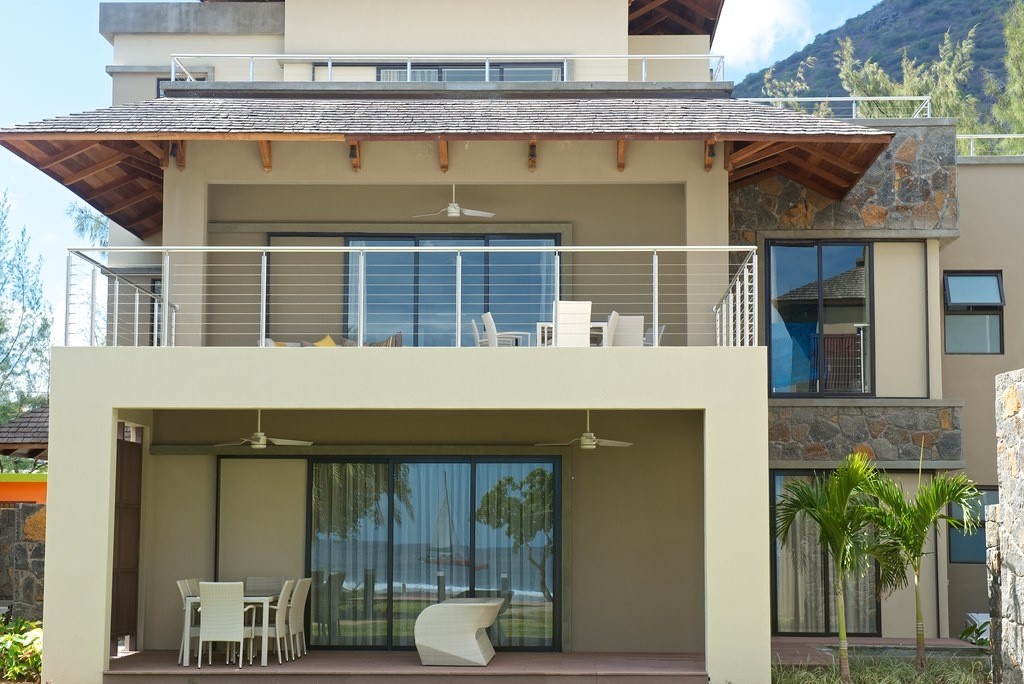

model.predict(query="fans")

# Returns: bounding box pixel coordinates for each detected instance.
[535,408,634,449]
[411,184,495,218]
[213,410,314,449]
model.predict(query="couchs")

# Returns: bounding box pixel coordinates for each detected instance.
[258,331,404,346]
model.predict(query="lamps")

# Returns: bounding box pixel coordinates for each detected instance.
[527,146,537,158]
[349,145,358,158]
[707,145,717,158]
[170,144,179,157]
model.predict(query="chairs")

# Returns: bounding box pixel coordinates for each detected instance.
[471,301,666,346]
[175,576,312,668]
[413,596,506,666]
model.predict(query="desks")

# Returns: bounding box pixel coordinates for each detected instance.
[184,593,280,666]
[536,322,608,346]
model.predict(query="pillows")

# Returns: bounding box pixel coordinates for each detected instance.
[301,335,395,346]
